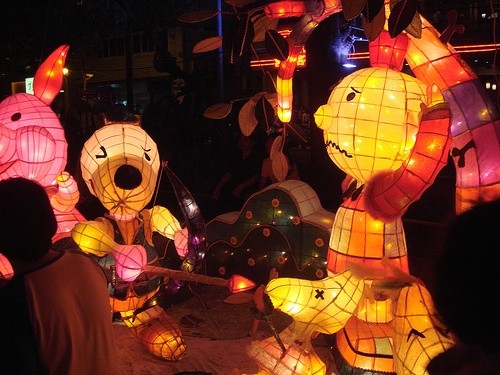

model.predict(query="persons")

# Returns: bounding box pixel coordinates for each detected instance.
[421,197,500,375]
[210,136,264,206]
[256,133,303,186]
[0,176,119,374]
